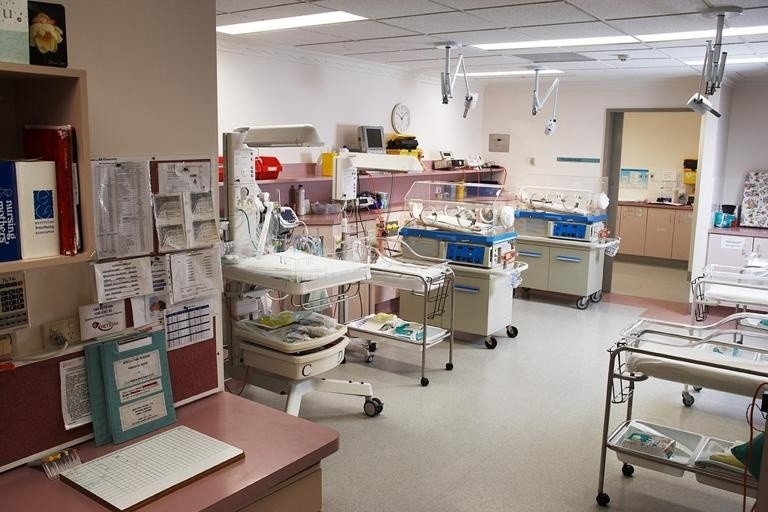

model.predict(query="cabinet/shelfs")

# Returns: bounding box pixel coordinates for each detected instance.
[512,239,605,297]
[672,210,694,261]
[706,227,768,310]
[645,207,676,259]
[616,204,621,254]
[398,264,515,338]
[218,159,508,221]
[0,64,97,273]
[621,206,648,255]
[683,169,696,206]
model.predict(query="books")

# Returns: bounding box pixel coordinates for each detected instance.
[82,321,178,448]
[0,120,86,258]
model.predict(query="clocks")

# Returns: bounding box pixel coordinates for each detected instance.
[391,100,412,134]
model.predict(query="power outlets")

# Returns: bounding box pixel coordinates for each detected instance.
[42,318,80,347]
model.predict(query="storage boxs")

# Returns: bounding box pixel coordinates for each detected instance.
[610,416,704,481]
[694,433,758,499]
[386,319,453,352]
[348,309,403,343]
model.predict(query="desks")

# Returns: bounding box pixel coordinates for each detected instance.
[1,389,340,511]
